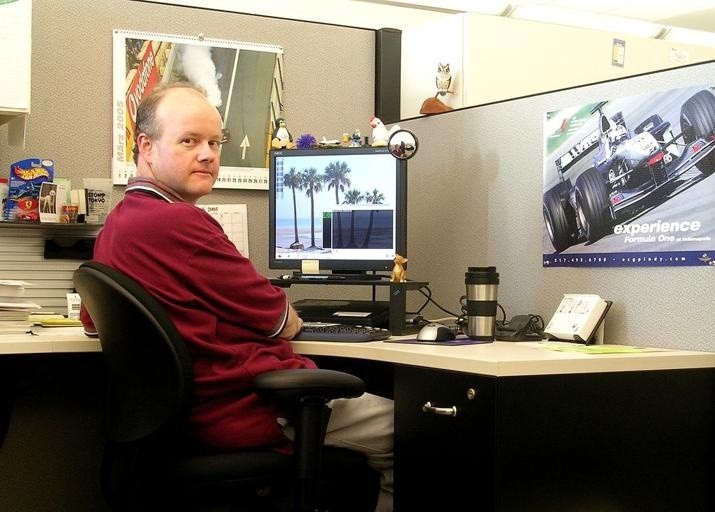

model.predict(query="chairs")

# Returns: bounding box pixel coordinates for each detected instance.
[73,261,381,512]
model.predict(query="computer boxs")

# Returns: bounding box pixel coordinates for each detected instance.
[290,298,389,330]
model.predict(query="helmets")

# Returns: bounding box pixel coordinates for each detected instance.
[609,125,627,143]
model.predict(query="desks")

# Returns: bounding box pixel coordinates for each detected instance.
[1,318,714,512]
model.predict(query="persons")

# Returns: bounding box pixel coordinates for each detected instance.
[79,82,395,512]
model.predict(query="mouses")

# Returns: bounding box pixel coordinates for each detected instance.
[417,321,456,341]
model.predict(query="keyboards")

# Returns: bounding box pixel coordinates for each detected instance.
[294,321,393,343]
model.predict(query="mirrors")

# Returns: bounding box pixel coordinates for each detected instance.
[374,1,715,125]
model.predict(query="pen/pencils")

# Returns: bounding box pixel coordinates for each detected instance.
[295,276,346,279]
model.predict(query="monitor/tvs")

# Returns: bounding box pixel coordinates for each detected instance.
[268,146,408,280]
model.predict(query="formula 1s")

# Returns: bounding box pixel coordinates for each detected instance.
[543,90,715,252]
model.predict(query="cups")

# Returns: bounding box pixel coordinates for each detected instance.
[464,267,500,341]
[62,204,79,224]
